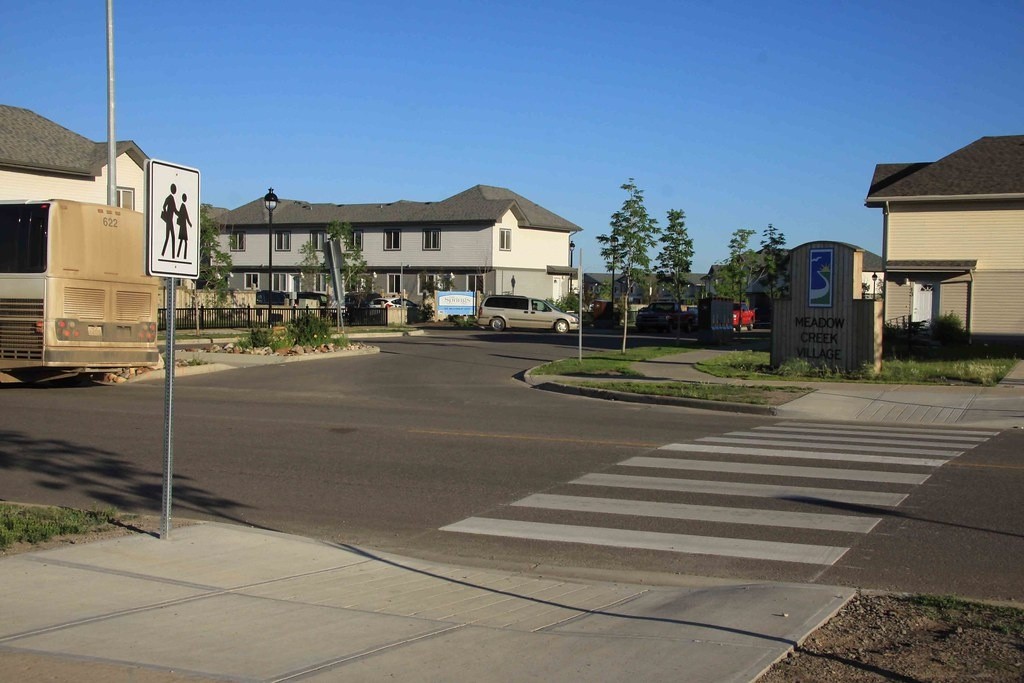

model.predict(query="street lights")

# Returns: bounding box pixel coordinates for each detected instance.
[609,235,620,311]
[871,271,878,300]
[263,188,282,329]
[568,240,575,294]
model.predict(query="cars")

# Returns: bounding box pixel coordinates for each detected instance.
[730,302,756,332]
[257,289,288,322]
[346,291,382,320]
[296,290,335,318]
[635,302,698,333]
[367,297,418,320]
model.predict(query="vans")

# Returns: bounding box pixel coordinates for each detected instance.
[477,295,580,334]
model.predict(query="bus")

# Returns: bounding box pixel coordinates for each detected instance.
[0,196,163,386]
